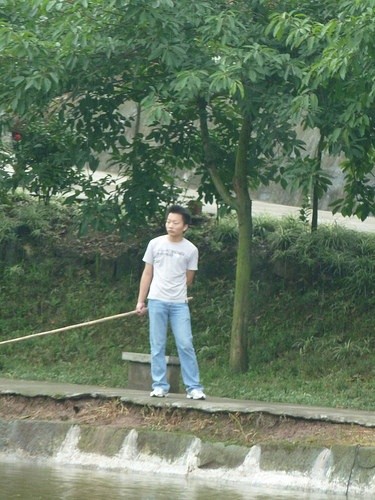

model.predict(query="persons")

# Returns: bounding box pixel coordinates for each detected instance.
[136,204,207,400]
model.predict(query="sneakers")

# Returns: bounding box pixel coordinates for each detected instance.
[185,389,207,400]
[149,386,169,398]
